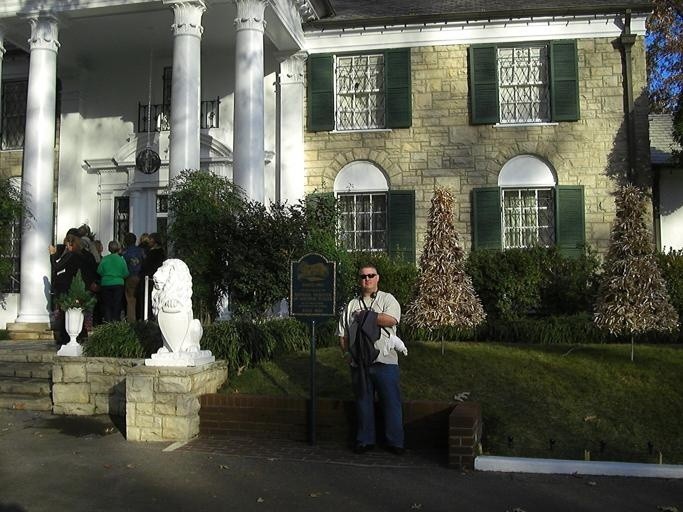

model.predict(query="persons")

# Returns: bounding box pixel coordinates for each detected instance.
[331,264,414,459]
[43,222,167,349]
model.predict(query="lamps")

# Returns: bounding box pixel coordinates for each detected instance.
[135,46,161,175]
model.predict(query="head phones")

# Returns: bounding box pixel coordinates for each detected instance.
[359,290,379,300]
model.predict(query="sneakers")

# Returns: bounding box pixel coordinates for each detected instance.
[389,444,406,456]
[355,443,373,455]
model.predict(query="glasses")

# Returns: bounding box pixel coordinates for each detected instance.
[360,274,376,279]
